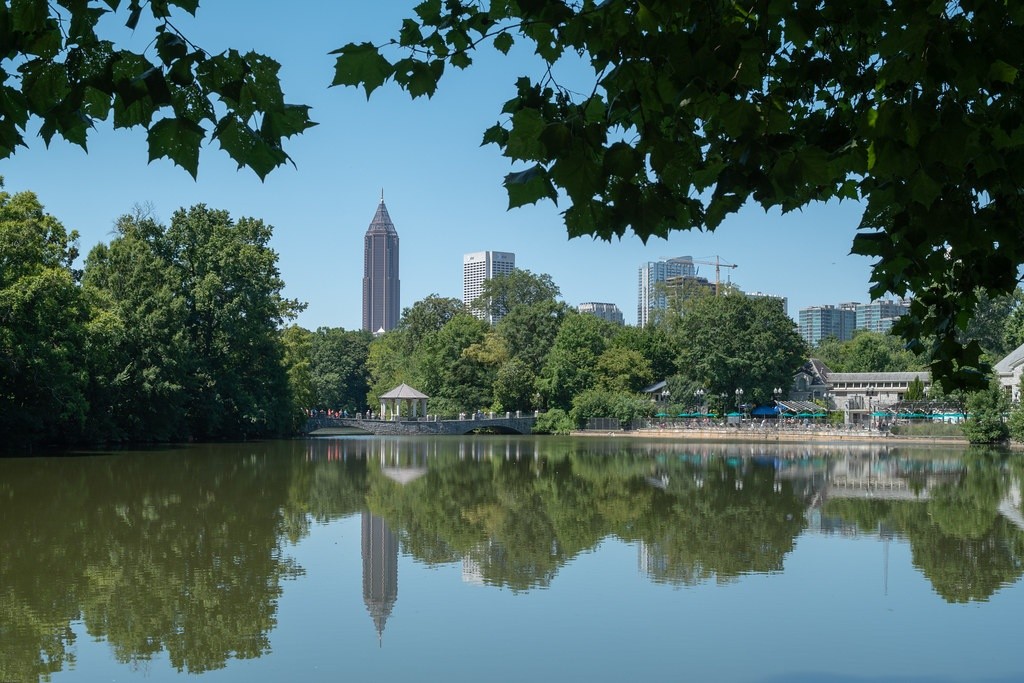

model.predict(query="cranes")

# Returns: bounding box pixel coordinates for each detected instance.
[670,253,738,297]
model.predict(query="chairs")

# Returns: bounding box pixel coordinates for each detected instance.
[648,418,865,434]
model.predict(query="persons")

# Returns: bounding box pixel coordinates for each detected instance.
[365,409,375,419]
[300,406,349,418]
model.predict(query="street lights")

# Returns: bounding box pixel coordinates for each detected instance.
[661,390,671,427]
[773,386,783,428]
[693,389,704,429]
[823,391,832,429]
[735,388,743,429]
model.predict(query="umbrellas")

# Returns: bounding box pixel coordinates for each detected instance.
[869,411,978,430]
[751,404,776,418]
[677,412,716,421]
[654,412,669,419]
[797,412,811,423]
[727,412,743,423]
[778,413,793,423]
[811,413,827,422]
